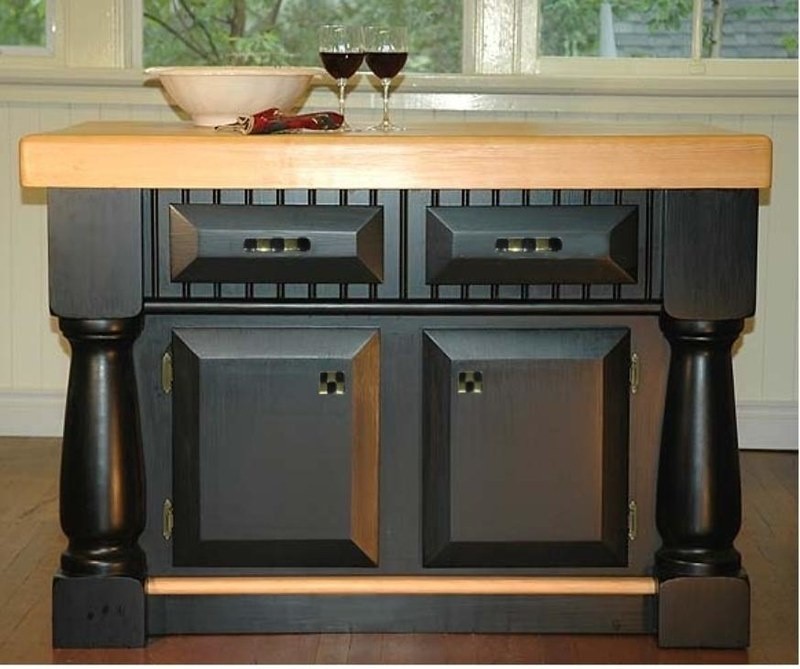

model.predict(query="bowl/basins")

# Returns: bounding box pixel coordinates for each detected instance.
[142,65,328,129]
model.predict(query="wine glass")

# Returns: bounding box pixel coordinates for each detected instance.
[365,24,409,134]
[316,23,365,134]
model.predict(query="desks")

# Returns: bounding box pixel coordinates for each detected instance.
[18,120,771,649]
